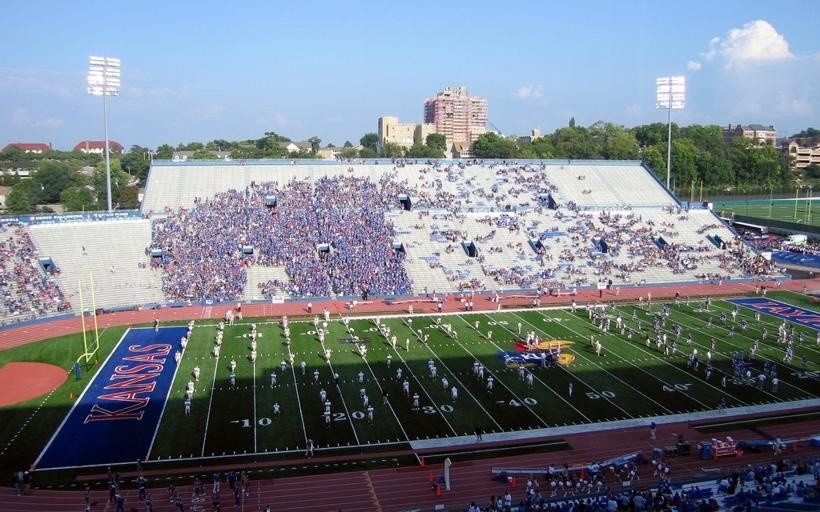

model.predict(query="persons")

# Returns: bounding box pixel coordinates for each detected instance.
[0,153,820,510]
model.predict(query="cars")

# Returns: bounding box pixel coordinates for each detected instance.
[747,232,762,241]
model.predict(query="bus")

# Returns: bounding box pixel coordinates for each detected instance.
[732,221,769,240]
[719,217,735,229]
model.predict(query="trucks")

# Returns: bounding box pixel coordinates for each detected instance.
[783,235,808,245]
